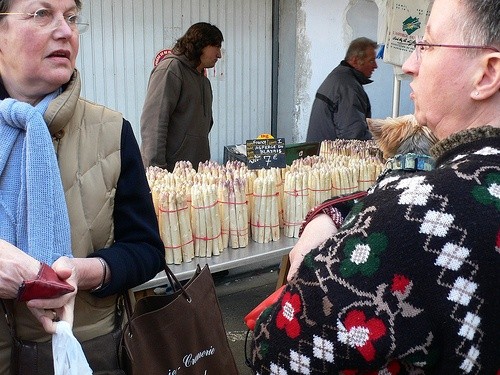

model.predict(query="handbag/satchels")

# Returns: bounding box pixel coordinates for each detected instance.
[8,324,123,375]
[118,263,241,375]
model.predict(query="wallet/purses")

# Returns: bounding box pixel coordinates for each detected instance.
[16,262,76,303]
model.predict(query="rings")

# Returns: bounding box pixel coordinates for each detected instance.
[51,309,58,320]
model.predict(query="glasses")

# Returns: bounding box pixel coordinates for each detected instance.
[0,8,90,36]
[412,35,500,62]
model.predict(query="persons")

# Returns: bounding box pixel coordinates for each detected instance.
[306,37,378,140]
[140,22,223,173]
[251,0,500,375]
[0,0,167,375]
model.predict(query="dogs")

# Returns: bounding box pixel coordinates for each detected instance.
[366,114,438,196]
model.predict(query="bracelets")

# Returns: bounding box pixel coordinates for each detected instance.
[87,257,106,293]
